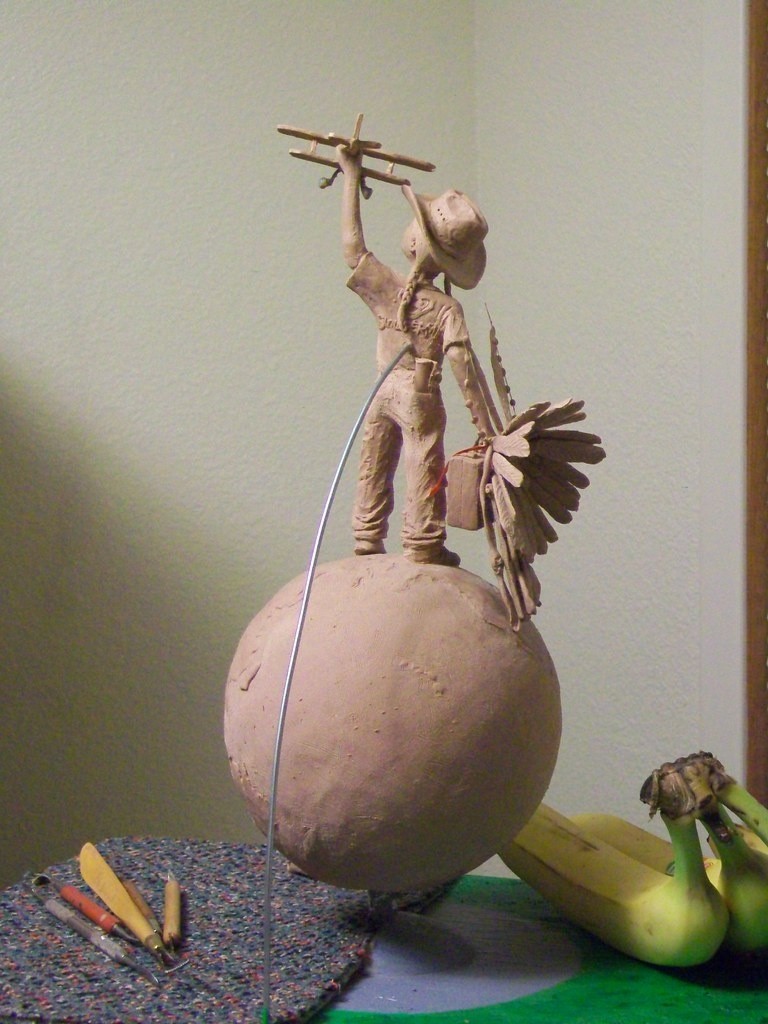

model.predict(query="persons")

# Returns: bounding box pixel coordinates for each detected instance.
[336,134,497,570]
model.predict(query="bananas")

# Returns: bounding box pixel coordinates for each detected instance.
[496,750,768,968]
